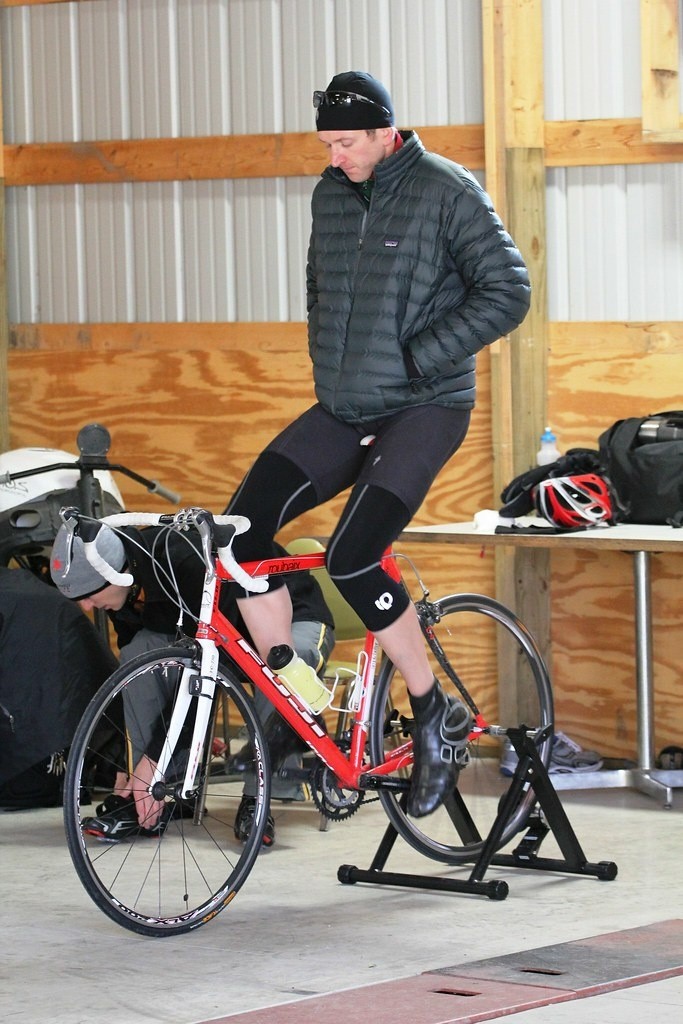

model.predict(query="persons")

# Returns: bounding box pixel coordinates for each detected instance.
[217,72,532,819]
[49,503,338,849]
[0,567,130,812]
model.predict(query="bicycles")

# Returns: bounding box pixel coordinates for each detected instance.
[52,505,556,938]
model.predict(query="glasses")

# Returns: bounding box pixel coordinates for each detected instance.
[312,91,388,114]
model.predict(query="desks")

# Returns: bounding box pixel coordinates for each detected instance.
[394,516,683,812]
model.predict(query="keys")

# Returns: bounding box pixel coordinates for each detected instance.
[53,754,63,775]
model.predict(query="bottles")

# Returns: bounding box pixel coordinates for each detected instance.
[536,427,561,467]
[267,643,330,712]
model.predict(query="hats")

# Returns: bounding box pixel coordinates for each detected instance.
[316,71,394,131]
[49,517,130,602]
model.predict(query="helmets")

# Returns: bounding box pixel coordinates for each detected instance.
[532,475,629,534]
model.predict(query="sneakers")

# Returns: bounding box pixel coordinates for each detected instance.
[234,800,275,848]
[231,710,327,772]
[76,811,166,840]
[499,732,602,778]
[402,681,470,819]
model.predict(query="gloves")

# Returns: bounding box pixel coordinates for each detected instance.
[500,454,591,518]
